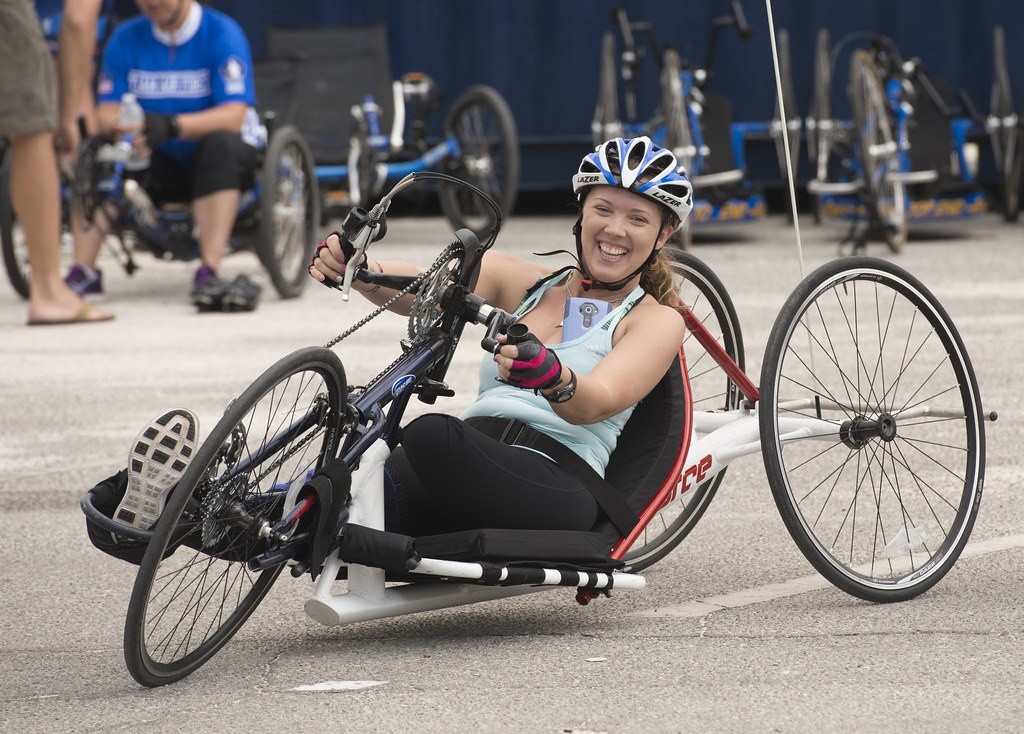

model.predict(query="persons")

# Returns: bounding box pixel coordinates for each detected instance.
[0,0,261,325]
[111,136,694,538]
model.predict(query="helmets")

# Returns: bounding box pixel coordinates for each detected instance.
[572,136,694,234]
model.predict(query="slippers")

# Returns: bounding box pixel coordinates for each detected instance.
[28,302,114,324]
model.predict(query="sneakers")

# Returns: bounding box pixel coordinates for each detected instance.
[111,407,200,544]
[65,264,104,297]
[195,276,263,311]
[190,266,218,293]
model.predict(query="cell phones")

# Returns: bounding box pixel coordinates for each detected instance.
[560,297,612,343]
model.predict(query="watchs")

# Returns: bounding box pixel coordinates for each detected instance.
[538,367,577,402]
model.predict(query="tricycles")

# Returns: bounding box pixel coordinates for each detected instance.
[78,170,998,691]
[589,0,1021,259]
[3,116,319,301]
[313,71,519,241]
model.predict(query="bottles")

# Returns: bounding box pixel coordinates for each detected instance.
[115,93,151,170]
